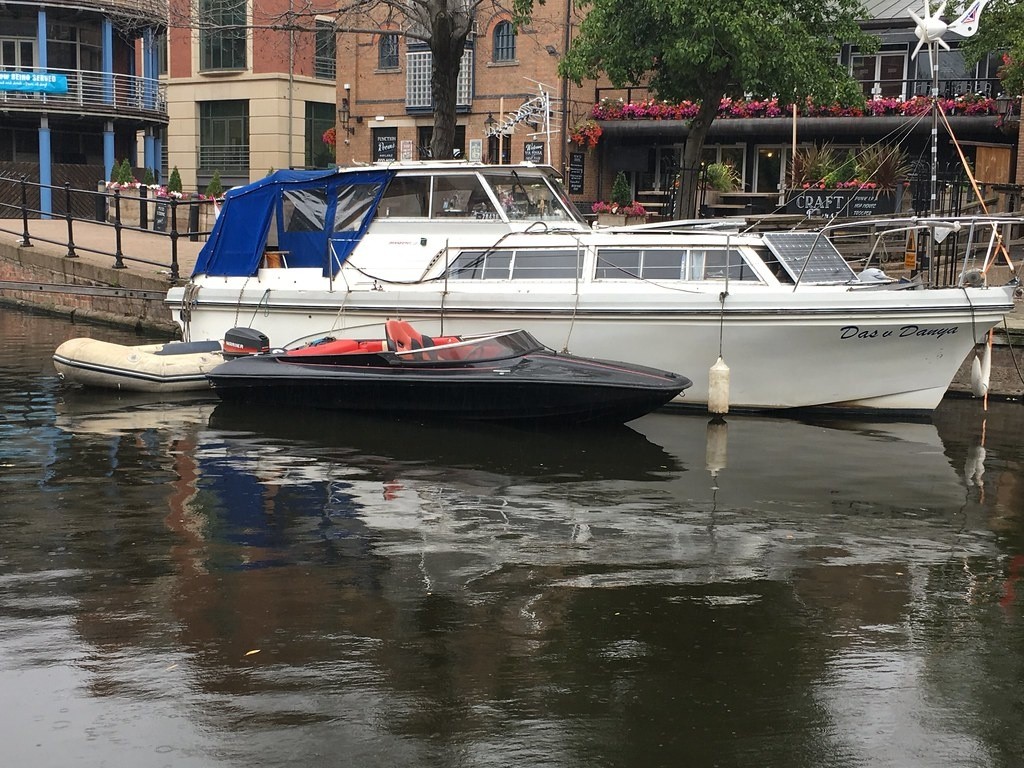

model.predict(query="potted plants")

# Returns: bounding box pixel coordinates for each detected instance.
[198,169,223,242]
[783,137,916,218]
[669,163,750,219]
[158,166,191,234]
[105,159,157,227]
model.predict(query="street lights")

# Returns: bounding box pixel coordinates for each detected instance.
[484,110,498,165]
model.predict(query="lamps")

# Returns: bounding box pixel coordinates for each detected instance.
[338,102,354,135]
[484,112,496,140]
[996,89,1013,127]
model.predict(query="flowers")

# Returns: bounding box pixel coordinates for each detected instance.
[802,181,910,191]
[571,93,1022,150]
[107,180,225,200]
[322,127,336,157]
[591,202,648,218]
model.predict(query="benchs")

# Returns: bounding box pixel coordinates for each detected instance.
[287,339,388,355]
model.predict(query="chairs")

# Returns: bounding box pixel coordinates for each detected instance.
[385,320,443,361]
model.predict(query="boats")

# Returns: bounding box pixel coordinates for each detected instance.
[52,334,225,393]
[53,89,1024,414]
[204,324,694,422]
